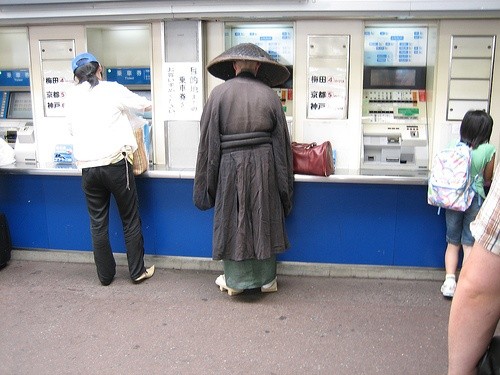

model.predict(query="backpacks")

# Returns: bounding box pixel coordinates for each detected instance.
[427,142,479,212]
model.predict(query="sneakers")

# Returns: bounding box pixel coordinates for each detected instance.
[440,282,457,297]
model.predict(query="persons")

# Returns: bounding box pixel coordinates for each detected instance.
[64,54,155,285]
[446,161,499,375]
[440,109,496,297]
[192,42,294,296]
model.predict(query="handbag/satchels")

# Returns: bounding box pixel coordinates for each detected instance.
[290,140,335,177]
[130,125,148,176]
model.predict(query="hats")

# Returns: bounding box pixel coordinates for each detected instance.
[71,53,100,72]
[206,42,290,87]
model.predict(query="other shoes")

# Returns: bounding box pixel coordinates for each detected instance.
[134,264,155,283]
[215,275,244,296]
[261,281,278,292]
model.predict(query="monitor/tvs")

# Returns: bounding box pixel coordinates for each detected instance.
[132,90,152,119]
[363,65,426,90]
[6,91,32,119]
